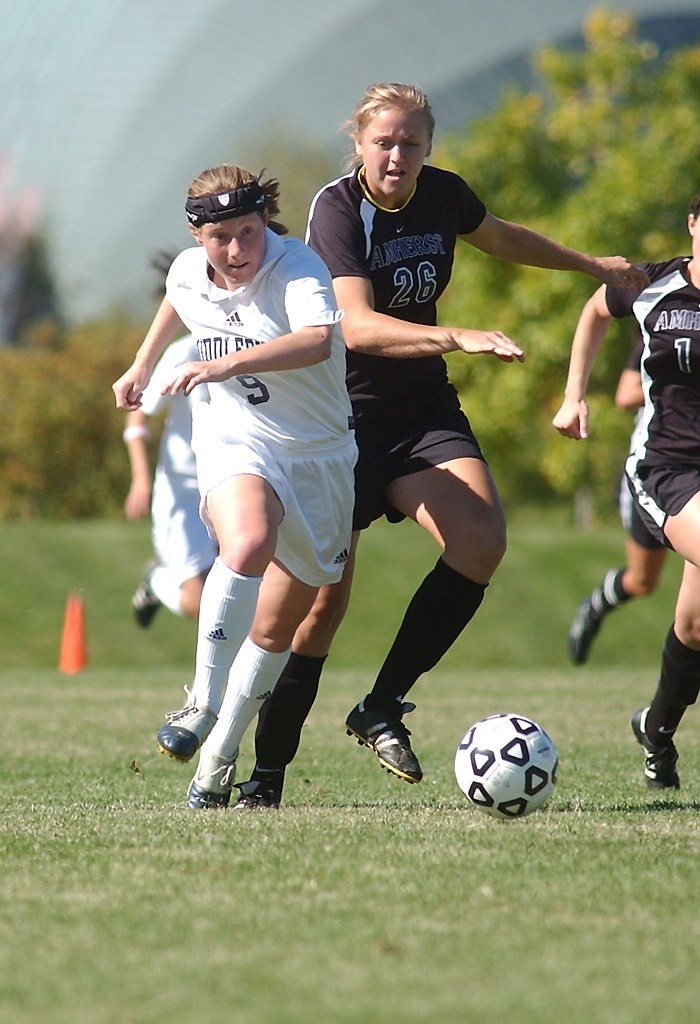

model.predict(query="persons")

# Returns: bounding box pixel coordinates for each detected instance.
[552,192,700,790]
[133,255,221,629]
[304,83,653,782]
[112,166,358,812]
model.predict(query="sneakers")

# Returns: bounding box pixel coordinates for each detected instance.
[156,684,218,764]
[187,744,240,810]
[631,708,680,790]
[567,595,602,667]
[345,702,422,783]
[131,560,160,628]
[233,764,285,810]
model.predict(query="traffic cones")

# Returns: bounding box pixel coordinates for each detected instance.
[54,591,89,674]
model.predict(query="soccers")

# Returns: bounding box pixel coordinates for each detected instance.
[454,712,561,819]
